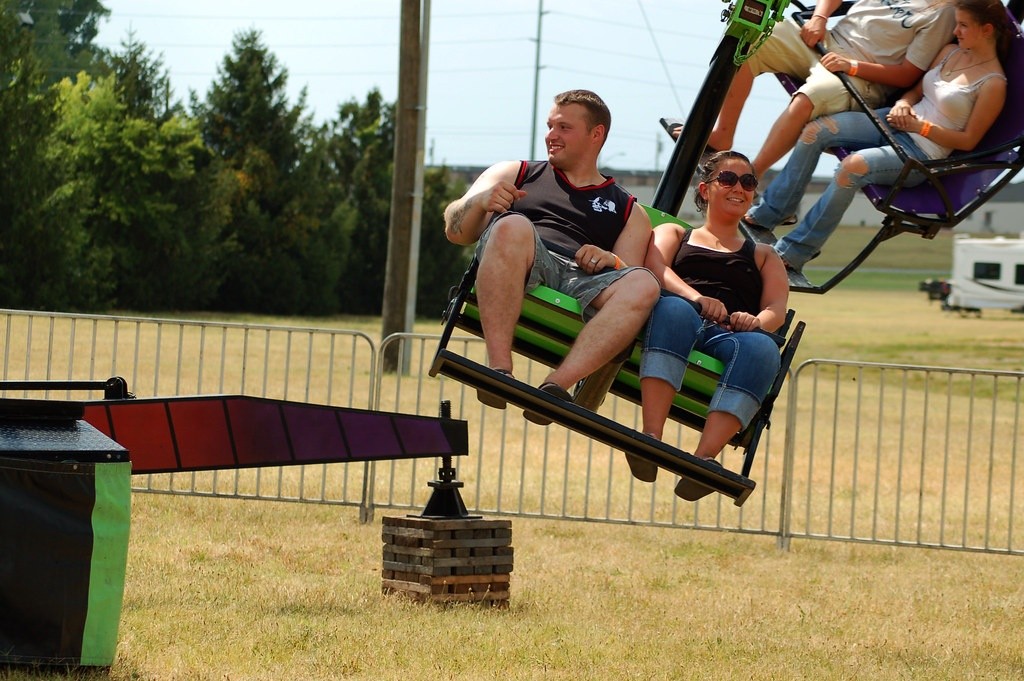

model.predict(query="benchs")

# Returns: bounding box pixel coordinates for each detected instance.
[778,0,1024,234]
[428,167,804,508]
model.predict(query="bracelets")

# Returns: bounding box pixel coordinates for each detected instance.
[920,120,932,138]
[809,15,829,22]
[848,58,859,77]
[611,252,621,270]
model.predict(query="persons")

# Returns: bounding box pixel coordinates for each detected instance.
[666,0,957,197]
[619,149,792,504]
[441,88,663,430]
[741,0,1013,274]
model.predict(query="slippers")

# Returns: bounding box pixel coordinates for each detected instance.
[477,369,511,410]
[744,205,798,231]
[674,460,724,503]
[624,434,658,483]
[778,251,823,271]
[522,382,572,425]
[667,122,720,153]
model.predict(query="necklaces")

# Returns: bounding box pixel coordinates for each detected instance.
[943,49,997,77]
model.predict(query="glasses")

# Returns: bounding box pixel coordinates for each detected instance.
[705,171,759,192]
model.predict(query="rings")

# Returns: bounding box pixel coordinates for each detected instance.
[590,259,597,265]
